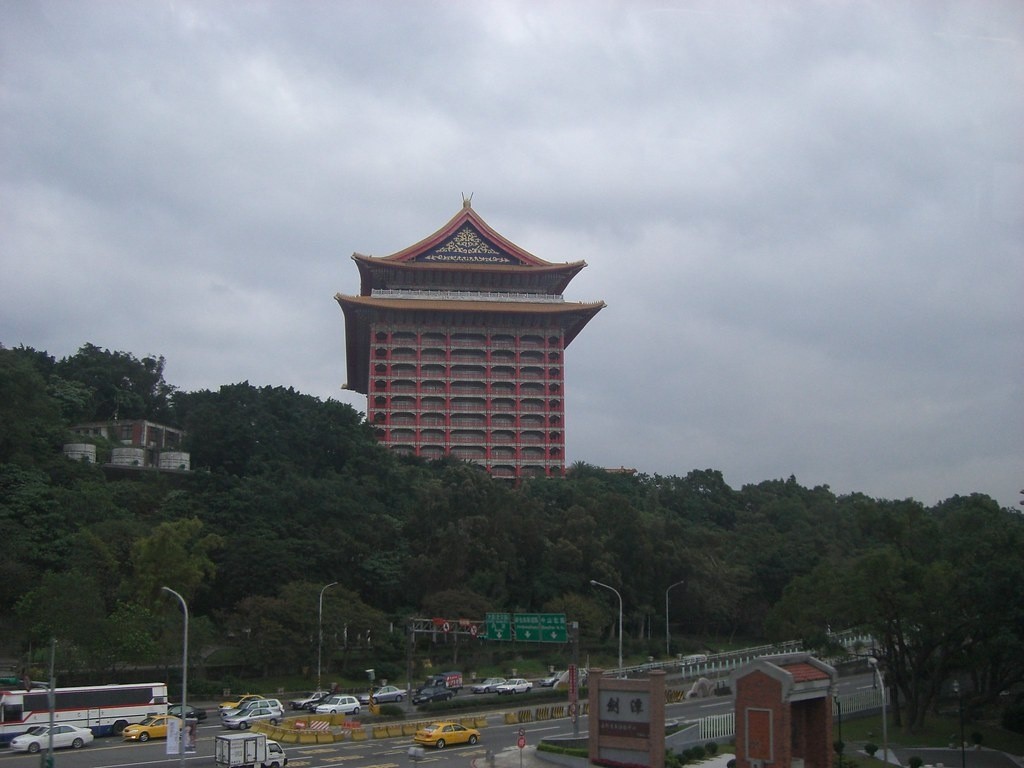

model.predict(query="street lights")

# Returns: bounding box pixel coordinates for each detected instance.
[832,686,843,768]
[666,580,684,659]
[590,580,623,678]
[867,658,888,764]
[316,581,340,691]
[161,586,188,766]
[953,680,965,768]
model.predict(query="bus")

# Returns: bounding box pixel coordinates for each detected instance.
[0,682,169,747]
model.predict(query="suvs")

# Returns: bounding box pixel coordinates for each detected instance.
[219,698,285,721]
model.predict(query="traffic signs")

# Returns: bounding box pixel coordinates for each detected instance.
[540,614,569,644]
[514,612,542,642]
[484,613,512,641]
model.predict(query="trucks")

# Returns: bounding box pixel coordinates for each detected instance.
[416,670,464,697]
[214,732,288,768]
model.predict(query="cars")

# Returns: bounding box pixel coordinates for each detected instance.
[315,695,361,716]
[221,707,283,730]
[9,723,94,754]
[471,677,507,694]
[217,694,265,714]
[287,691,330,710]
[539,670,566,687]
[303,692,350,713]
[495,678,533,696]
[122,715,181,743]
[167,703,208,725]
[411,687,454,705]
[358,685,407,705]
[412,723,481,750]
